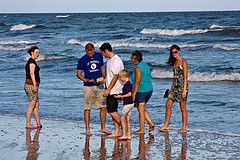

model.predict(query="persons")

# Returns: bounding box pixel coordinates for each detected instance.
[76,43,112,135]
[25,128,41,160]
[83,132,188,160]
[130,49,155,134]
[98,42,128,138]
[113,69,134,140]
[158,44,189,133]
[24,46,43,129]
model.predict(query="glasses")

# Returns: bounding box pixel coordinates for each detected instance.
[171,51,179,54]
